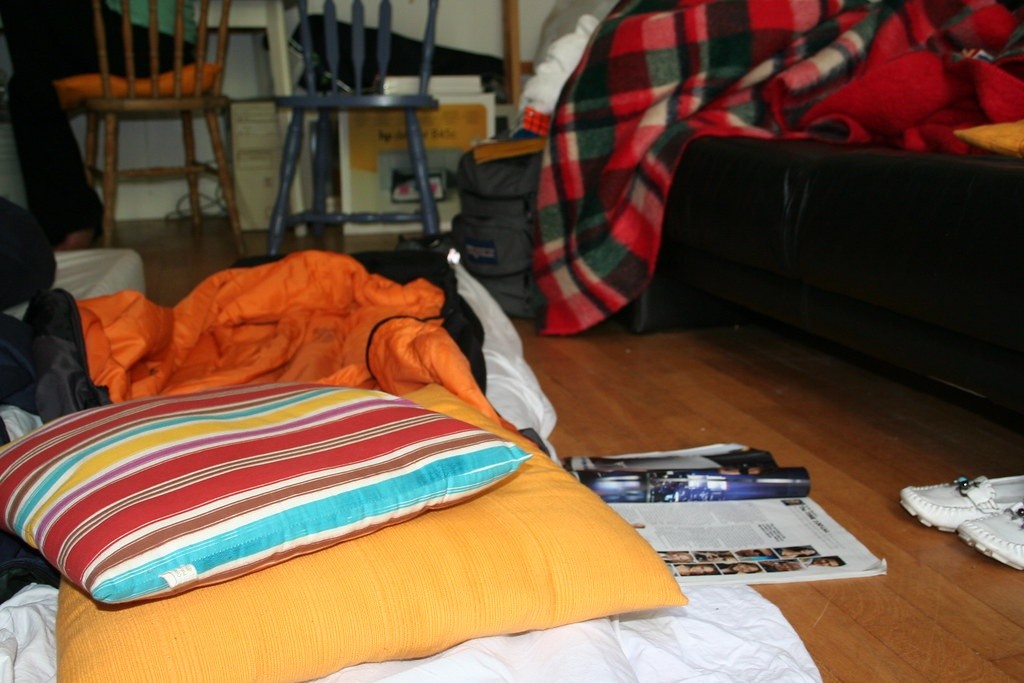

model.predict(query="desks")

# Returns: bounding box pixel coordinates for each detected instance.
[193,0,307,240]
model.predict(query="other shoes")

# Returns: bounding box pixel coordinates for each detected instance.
[900,475,1024,532]
[957,502,1024,571]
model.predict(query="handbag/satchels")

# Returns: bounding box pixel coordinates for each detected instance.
[449,148,541,319]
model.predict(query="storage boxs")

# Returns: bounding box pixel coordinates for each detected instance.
[336,95,497,236]
[221,99,281,232]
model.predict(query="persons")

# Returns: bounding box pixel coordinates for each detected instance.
[0,0,199,250]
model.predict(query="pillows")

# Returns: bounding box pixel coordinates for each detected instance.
[0,385,529,608]
[56,382,689,683]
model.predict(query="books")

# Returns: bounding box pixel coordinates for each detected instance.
[559,443,887,588]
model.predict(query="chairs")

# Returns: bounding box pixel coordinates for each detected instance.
[83,1,245,261]
[266,0,443,256]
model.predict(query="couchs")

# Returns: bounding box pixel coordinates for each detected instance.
[617,137,1024,426]
